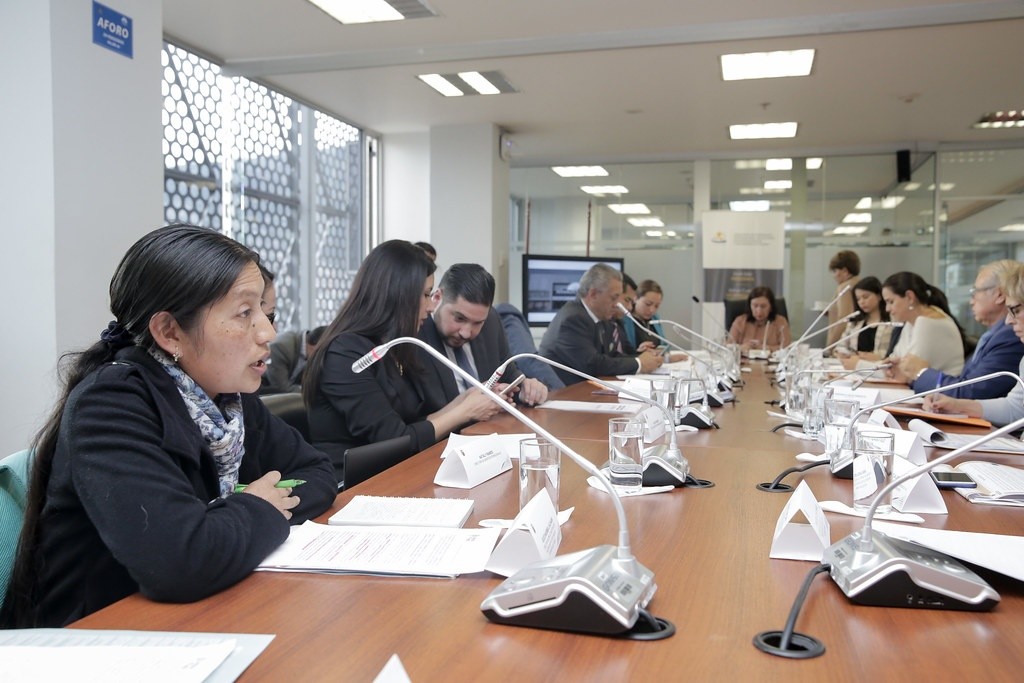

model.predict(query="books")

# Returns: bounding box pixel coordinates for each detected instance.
[934,461,1024,506]
[908,419,1024,451]
[328,495,481,530]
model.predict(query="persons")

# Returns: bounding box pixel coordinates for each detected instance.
[414,241,438,262]
[407,263,547,412]
[0,223,339,629]
[839,271,965,377]
[725,285,790,352]
[260,264,327,392]
[881,260,1024,399]
[827,252,906,359]
[496,302,566,392]
[540,263,662,386]
[610,275,671,352]
[923,263,1024,440]
[303,240,508,489]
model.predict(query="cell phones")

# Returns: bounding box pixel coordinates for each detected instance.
[750,340,759,348]
[931,472,977,488]
[660,344,670,356]
[500,374,525,395]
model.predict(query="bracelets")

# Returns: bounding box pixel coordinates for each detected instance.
[916,368,928,380]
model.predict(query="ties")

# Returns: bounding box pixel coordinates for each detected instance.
[597,320,606,354]
[970,330,992,362]
[612,322,623,354]
[452,346,476,389]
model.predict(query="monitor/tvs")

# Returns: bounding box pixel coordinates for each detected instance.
[522,254,624,327]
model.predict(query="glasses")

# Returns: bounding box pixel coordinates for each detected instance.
[265,314,276,325]
[1006,303,1023,319]
[969,284,997,297]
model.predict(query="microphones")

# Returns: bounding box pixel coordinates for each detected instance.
[772,286,904,424]
[617,296,741,428]
[352,336,657,635]
[485,353,691,487]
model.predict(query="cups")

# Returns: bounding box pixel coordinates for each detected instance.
[853,431,892,514]
[610,418,643,492]
[824,399,859,459]
[653,379,675,417]
[670,343,835,436]
[520,438,559,515]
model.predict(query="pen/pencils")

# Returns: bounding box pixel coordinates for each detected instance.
[930,372,944,413]
[848,347,859,355]
[234,478,307,493]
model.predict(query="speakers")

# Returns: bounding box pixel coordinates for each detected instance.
[897,148,911,183]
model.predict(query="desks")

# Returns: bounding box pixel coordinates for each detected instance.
[67,351,1024,683]
